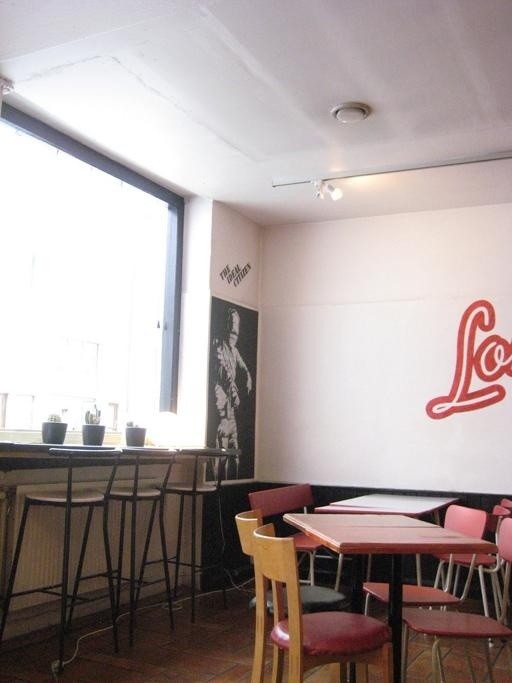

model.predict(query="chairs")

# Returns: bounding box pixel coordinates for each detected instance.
[1,448,123,674]
[137,449,231,623]
[68,450,178,646]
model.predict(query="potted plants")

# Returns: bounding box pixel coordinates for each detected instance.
[83,405,105,445]
[42,413,67,443]
[126,422,146,445]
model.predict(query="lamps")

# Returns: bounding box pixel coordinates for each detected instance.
[332,101,372,124]
[310,180,344,203]
